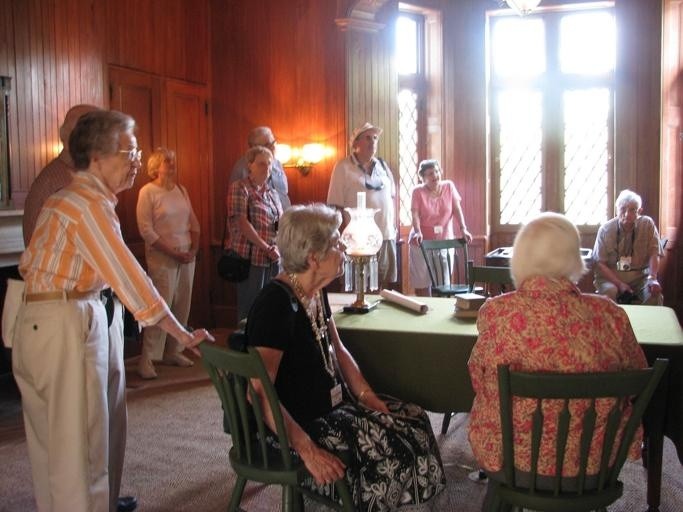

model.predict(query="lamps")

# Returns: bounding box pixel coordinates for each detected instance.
[506,0,541,18]
[340,191,383,312]
[274,143,324,175]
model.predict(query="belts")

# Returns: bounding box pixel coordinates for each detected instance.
[22,290,100,302]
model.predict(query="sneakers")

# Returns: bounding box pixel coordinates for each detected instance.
[136,362,157,379]
[172,351,194,367]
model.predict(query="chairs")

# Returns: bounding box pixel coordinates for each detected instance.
[481,359,670,512]
[198,343,354,511]
[467,260,509,295]
[422,239,483,296]
[595,239,668,304]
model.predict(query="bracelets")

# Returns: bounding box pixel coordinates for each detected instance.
[358,388,371,401]
[459,226,466,230]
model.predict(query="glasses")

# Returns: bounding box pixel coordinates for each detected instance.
[365,182,384,190]
[119,148,143,161]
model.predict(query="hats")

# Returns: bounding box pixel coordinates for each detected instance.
[352,122,382,142]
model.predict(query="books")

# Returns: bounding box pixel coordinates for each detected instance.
[452,291,488,319]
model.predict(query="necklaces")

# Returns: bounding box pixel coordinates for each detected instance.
[288,272,335,377]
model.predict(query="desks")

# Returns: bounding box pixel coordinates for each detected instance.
[484,246,594,297]
[239,290,682,511]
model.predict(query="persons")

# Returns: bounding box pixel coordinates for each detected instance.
[225,126,291,211]
[321,122,399,292]
[589,188,666,305]
[19,103,138,511]
[9,107,217,511]
[406,159,472,297]
[133,148,201,379]
[223,147,285,322]
[242,201,447,512]
[466,210,650,474]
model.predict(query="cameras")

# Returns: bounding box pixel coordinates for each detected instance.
[616,258,633,271]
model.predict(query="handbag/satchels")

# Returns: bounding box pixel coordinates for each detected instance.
[2,279,25,349]
[217,249,250,283]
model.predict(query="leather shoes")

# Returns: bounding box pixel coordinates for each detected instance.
[117,496,137,512]
